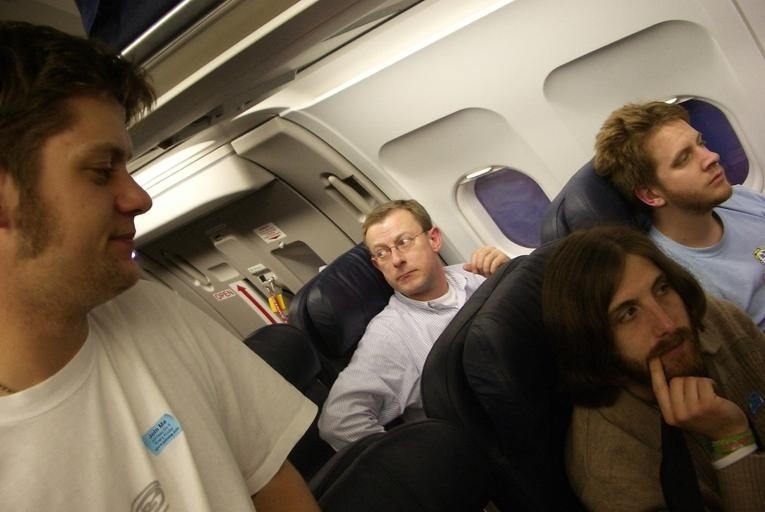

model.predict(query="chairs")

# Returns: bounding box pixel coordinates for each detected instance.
[538,149,647,248]
[240,324,325,479]
[289,238,394,374]
[419,251,576,414]
[316,415,581,511]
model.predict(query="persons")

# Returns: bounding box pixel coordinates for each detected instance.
[538,223,765,509]
[317,199,515,454]
[0,17,326,512]
[594,100,765,337]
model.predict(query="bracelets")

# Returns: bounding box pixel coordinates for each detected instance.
[710,437,756,459]
[712,441,759,471]
[710,428,754,447]
